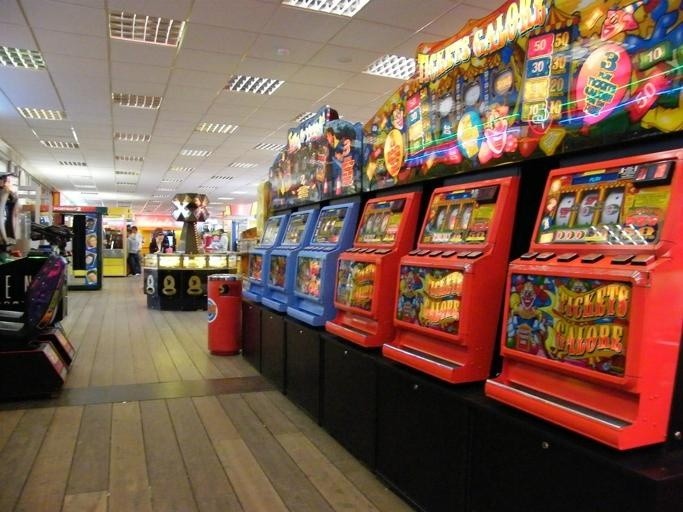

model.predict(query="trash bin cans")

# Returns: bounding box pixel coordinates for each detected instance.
[207,274,242,356]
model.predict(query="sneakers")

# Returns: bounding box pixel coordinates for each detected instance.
[128,272,141,277]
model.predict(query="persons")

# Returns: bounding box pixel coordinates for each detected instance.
[85,254,94,265]
[215,229,228,252]
[86,218,95,230]
[106,240,116,249]
[87,235,97,247]
[132,226,142,274]
[152,229,169,248]
[127,229,139,276]
[149,232,167,254]
[203,229,212,248]
[87,271,97,282]
